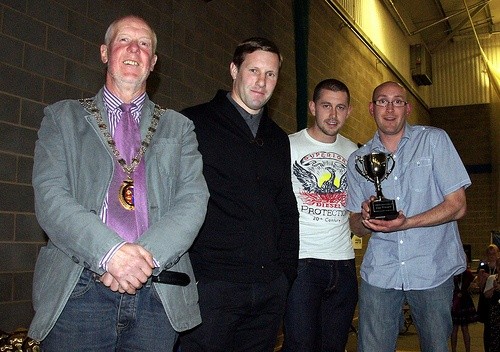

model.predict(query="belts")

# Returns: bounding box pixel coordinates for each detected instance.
[151,270,191,287]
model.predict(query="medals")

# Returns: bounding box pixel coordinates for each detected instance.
[119,179,135,210]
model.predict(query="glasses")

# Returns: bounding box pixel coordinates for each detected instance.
[373,99,407,107]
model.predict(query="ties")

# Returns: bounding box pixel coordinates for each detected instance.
[105,102,149,245]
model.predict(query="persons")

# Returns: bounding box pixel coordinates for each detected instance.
[346,81,471,352]
[173,37,299,352]
[450,244,500,352]
[280,79,360,352]
[27,15,210,352]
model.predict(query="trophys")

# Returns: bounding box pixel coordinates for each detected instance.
[355,152,399,221]
